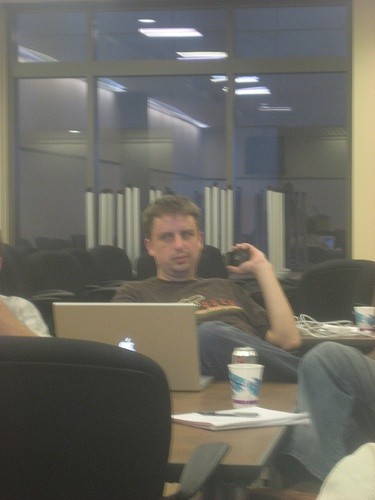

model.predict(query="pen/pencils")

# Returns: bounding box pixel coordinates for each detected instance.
[194,409,259,418]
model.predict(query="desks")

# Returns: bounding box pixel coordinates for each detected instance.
[171,381,295,500]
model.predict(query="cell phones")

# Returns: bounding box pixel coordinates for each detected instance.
[222,250,251,266]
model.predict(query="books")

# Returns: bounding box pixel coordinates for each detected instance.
[171,406,311,430]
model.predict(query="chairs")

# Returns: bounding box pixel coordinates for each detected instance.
[0,336,171,500]
[300,259,375,327]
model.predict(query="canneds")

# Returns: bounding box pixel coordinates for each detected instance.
[231,346,257,364]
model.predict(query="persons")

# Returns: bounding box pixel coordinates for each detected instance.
[308,215,346,263]
[110,196,303,383]
[272,341,375,500]
[0,242,51,337]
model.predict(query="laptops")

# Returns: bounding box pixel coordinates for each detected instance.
[52,302,214,392]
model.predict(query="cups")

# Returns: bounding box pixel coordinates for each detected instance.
[353,305,375,335]
[226,362,265,409]
